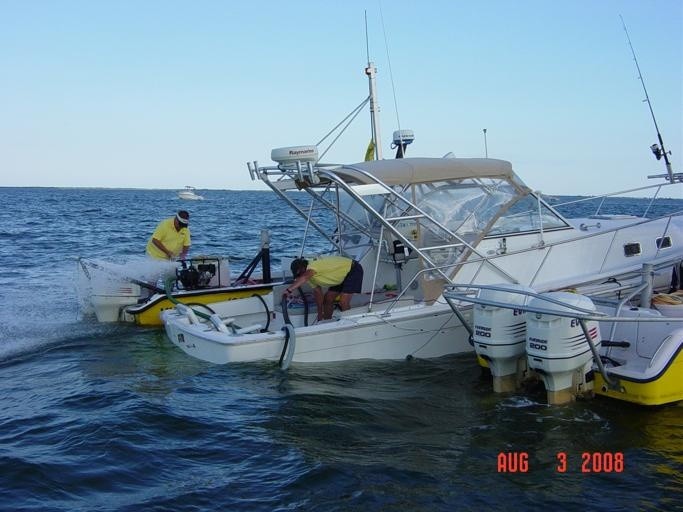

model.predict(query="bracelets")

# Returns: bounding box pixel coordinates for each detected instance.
[286,288,292,293]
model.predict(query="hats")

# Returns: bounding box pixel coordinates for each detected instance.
[176,213,189,228]
[291,259,304,280]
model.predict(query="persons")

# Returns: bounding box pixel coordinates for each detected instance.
[143,209,190,298]
[280,256,364,322]
